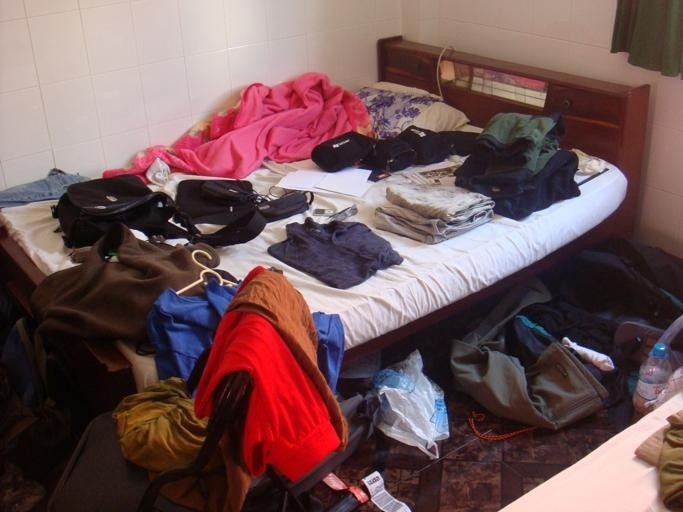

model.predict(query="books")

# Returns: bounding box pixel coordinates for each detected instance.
[353,162,392,182]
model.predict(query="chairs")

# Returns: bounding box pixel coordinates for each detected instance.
[39,266,324,511]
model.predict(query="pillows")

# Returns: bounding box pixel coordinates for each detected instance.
[355,81,472,143]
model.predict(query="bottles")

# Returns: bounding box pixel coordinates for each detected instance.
[631,342,670,411]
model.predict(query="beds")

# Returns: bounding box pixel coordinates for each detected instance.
[1,34,652,438]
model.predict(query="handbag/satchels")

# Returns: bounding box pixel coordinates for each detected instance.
[254,183,316,220]
[173,179,255,225]
[50,173,174,250]
